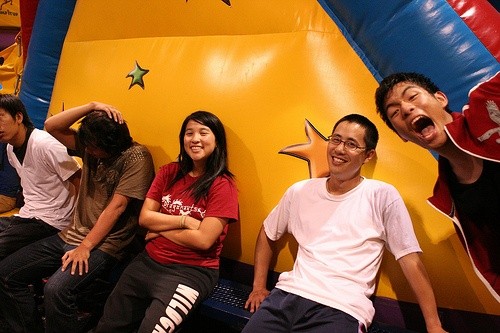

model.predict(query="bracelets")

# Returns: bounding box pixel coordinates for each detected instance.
[179,216,185,229]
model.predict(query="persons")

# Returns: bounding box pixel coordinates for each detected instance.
[239,114,449,333]
[375,70,500,304]
[95,110,238,333]
[0,94,82,261]
[0,102,156,333]
[0,141,19,213]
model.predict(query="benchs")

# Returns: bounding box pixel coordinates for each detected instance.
[99,241,500,333]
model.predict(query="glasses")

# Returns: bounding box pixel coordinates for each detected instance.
[328,135,371,151]
